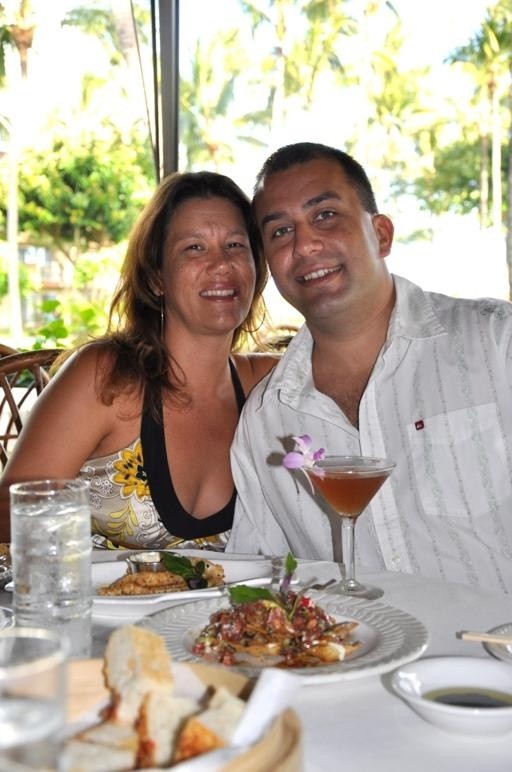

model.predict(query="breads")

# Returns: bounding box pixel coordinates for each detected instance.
[54,624,247,772]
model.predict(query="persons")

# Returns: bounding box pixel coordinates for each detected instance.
[0,172,276,551]
[227,143,510,597]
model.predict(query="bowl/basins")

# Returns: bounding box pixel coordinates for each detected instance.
[125,551,168,574]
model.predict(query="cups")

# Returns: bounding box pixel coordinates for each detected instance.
[7,477,93,628]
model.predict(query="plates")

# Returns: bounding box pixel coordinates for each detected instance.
[4,553,297,607]
[389,654,512,731]
[7,659,304,772]
[130,583,429,686]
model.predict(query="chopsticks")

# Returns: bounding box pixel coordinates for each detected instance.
[456,629,512,649]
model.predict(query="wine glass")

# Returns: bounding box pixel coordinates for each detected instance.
[304,453,396,601]
